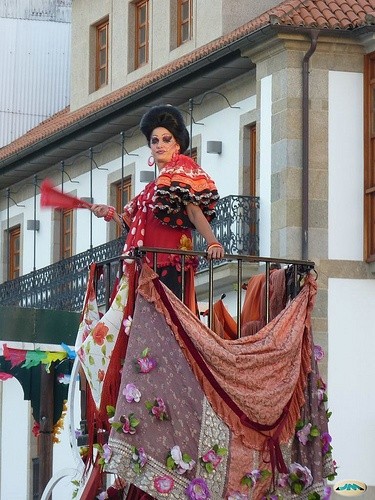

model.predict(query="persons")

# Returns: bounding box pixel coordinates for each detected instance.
[91,105,224,322]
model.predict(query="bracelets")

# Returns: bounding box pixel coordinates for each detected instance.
[103,205,116,222]
[206,242,223,250]
